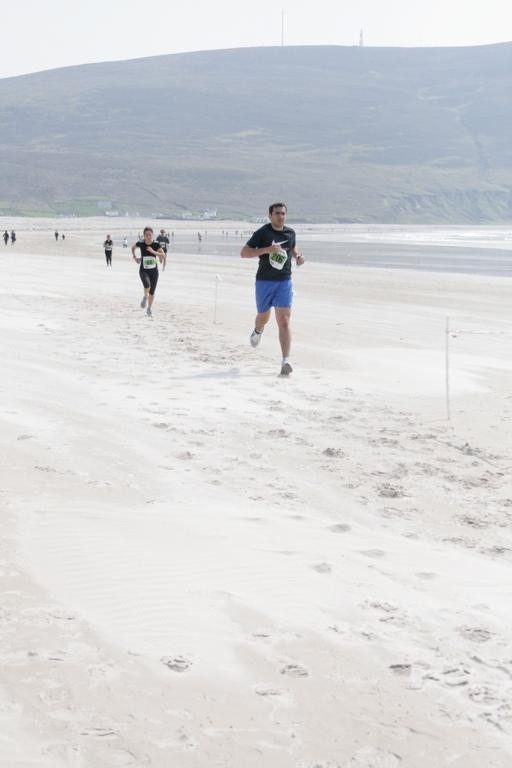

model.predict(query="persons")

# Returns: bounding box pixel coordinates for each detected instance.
[2,228,171,271]
[239,201,307,375]
[131,228,165,319]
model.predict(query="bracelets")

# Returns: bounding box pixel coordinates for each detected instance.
[294,252,301,260]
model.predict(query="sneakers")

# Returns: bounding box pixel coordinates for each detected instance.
[281,363,293,375]
[250,330,262,347]
[141,296,147,307]
